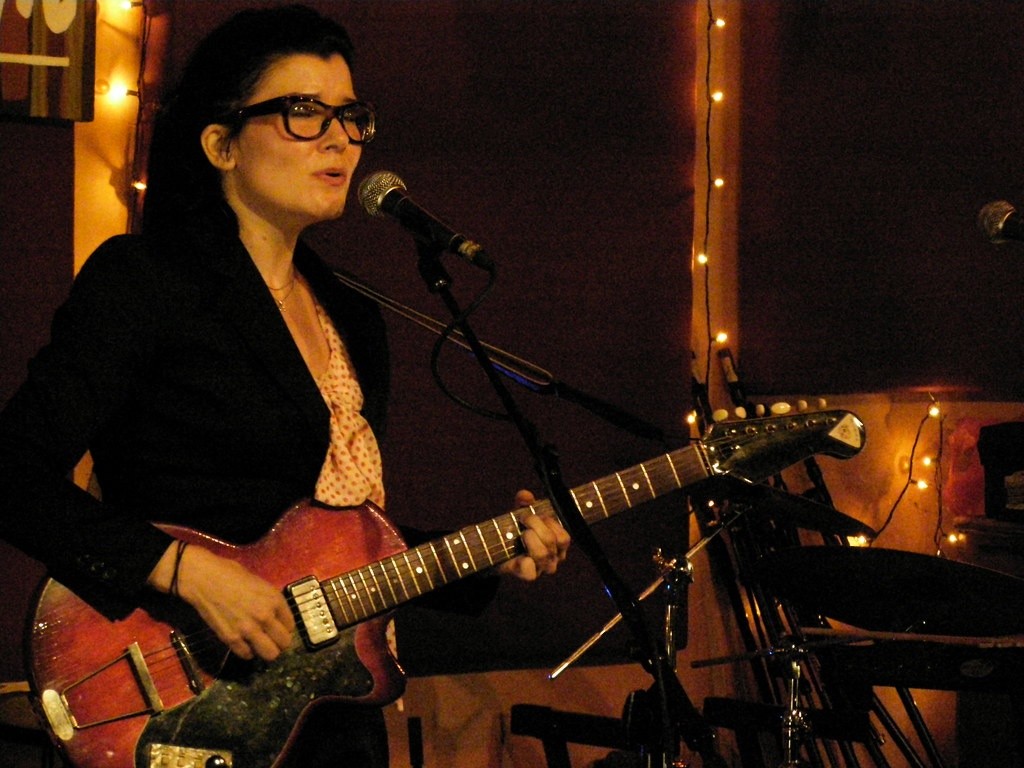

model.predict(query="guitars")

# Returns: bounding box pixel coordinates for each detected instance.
[27,397,869,768]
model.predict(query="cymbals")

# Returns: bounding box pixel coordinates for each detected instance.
[751,544,1024,639]
[718,472,880,541]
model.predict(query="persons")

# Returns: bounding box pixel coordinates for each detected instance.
[0,6,571,768]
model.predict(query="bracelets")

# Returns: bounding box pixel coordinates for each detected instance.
[169,541,188,596]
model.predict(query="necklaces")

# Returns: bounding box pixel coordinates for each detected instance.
[268,281,295,312]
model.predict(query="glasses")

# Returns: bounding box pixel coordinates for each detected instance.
[219,95,378,145]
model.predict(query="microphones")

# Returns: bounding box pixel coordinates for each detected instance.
[356,170,495,273]
[976,201,1024,242]
